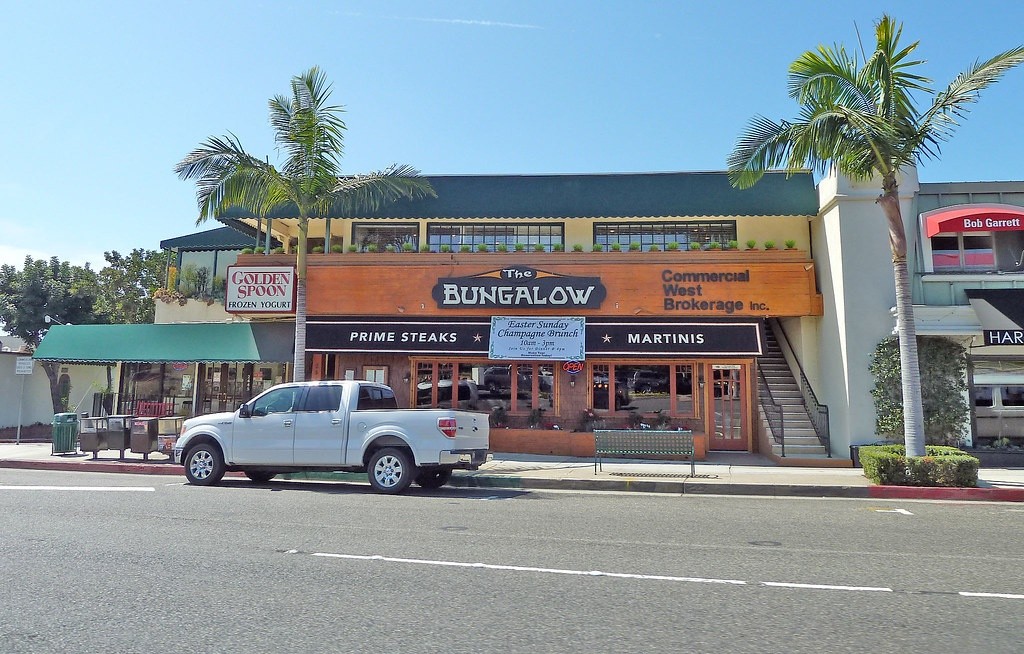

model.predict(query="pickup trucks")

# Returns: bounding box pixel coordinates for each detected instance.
[174,382,491,494]
[416,381,507,427]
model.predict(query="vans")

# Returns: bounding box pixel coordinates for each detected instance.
[632,371,687,394]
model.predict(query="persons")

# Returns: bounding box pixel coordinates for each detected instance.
[489,383,505,409]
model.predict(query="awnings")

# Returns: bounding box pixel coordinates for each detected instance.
[926,207,1024,238]
[31,322,293,364]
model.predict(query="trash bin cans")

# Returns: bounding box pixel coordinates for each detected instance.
[51,412,78,452]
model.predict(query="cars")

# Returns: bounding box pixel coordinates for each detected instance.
[515,370,554,390]
[548,382,629,413]
[483,367,545,394]
[713,381,734,398]
[138,375,182,398]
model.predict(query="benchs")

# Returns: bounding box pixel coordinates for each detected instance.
[594,429,695,477]
[128,400,174,417]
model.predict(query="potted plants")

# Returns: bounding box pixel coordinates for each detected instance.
[241,240,798,255]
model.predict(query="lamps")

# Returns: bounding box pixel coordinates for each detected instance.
[698,378,706,389]
[403,372,411,384]
[570,375,576,387]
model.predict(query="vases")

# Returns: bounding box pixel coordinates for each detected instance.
[489,427,705,460]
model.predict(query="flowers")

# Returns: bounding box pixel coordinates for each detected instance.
[491,408,673,433]
